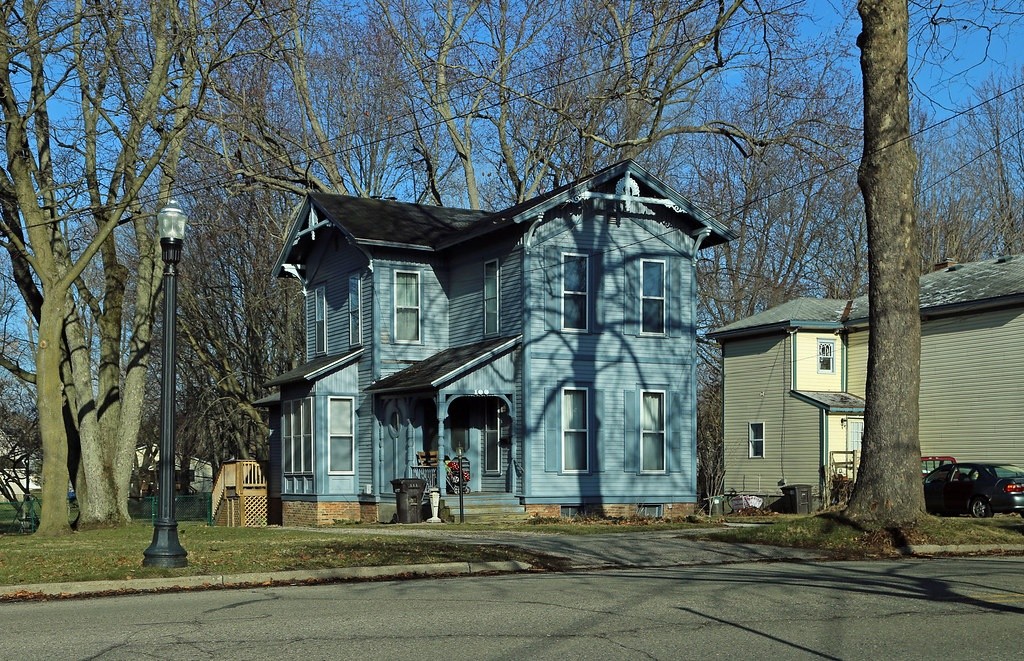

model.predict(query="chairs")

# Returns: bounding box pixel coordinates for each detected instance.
[418,450,438,478]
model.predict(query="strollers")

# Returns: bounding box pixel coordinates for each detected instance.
[444,462,471,495]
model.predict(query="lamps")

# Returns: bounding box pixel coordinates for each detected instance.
[455,447,463,457]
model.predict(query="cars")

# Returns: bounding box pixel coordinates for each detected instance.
[923,461,1024,518]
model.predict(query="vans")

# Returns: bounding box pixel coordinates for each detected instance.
[921,455,958,479]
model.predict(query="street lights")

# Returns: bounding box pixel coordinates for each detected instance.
[142,196,191,570]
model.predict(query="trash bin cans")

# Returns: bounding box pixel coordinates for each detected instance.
[780,484,813,514]
[390,478,427,524]
